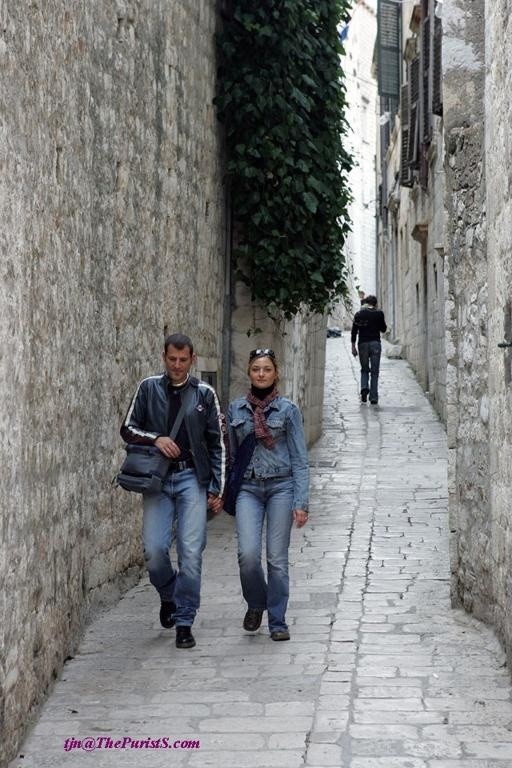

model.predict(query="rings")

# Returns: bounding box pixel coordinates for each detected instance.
[304,516,307,520]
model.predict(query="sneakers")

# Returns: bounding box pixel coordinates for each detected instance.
[361,390,378,405]
[158,601,174,629]
[271,630,292,641]
[242,608,264,630]
[174,625,197,648]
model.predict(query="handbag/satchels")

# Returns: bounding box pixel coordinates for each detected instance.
[220,433,258,517]
[117,438,174,493]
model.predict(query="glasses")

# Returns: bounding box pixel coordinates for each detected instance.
[248,347,276,363]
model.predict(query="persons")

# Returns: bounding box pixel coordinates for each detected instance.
[120,333,229,649]
[351,294,387,404]
[223,347,311,642]
[359,291,367,309]
